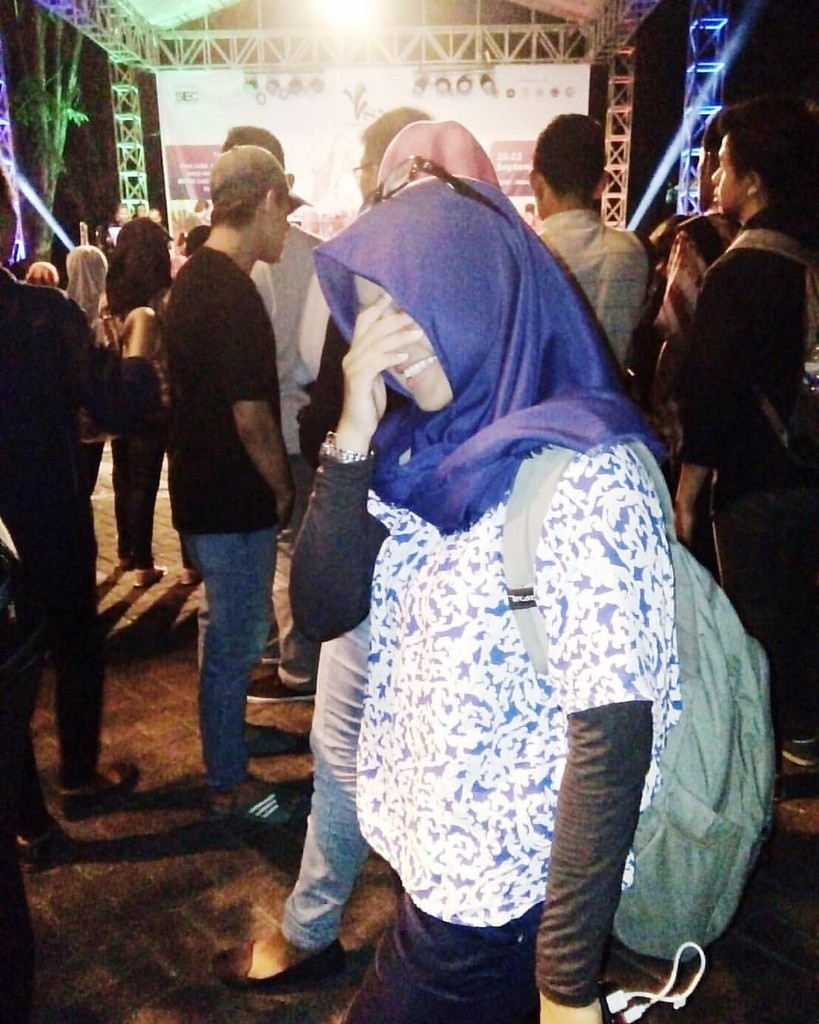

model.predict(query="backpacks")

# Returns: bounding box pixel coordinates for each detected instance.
[503,430,775,962]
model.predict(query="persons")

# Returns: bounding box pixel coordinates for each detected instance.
[521,110,658,375]
[670,92,819,804]
[0,538,40,1021]
[164,143,290,824]
[27,106,819,697]
[0,167,154,854]
[289,160,674,1024]
[206,121,502,995]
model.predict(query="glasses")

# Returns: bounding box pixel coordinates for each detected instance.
[357,154,514,227]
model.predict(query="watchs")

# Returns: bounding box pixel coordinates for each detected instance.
[317,431,367,462]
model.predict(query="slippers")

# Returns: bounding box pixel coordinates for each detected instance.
[131,569,163,588]
[178,569,204,585]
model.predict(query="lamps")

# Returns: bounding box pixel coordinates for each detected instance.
[412,73,494,96]
[244,77,324,104]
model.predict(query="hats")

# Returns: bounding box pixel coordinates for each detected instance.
[209,144,312,216]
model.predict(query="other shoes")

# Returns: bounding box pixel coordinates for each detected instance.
[774,740,818,766]
[211,937,343,991]
[119,558,135,570]
[208,780,289,826]
[265,620,279,648]
[241,722,294,757]
[245,669,316,704]
[61,757,140,819]
[17,816,66,864]
